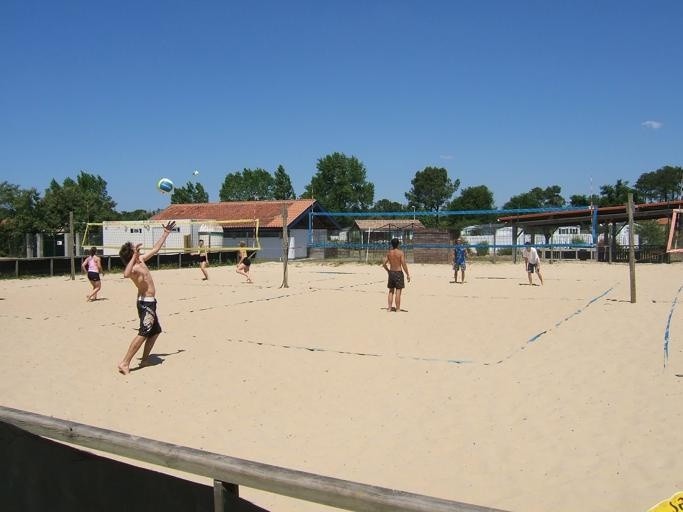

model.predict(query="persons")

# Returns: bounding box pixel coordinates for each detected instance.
[449,237,470,283]
[197,239,208,281]
[116,220,176,377]
[522,242,543,286]
[234,240,252,283]
[82,246,104,302]
[382,238,410,313]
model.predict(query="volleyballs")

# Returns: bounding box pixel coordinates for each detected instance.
[192,170,198,178]
[157,178,173,193]
